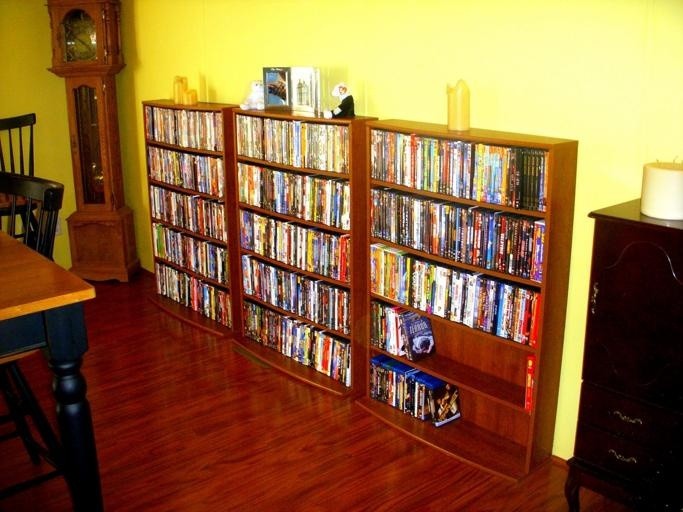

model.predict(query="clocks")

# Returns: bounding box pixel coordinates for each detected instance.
[45,0,139,283]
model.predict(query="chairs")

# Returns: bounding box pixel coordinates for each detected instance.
[0,171,64,498]
[0,113,38,242]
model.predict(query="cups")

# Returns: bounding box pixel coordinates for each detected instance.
[173,76,197,106]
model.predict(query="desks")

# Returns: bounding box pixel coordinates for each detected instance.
[0,230,103,512]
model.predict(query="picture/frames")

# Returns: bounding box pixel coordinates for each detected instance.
[263,67,290,115]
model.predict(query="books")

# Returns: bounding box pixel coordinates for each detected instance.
[145,106,231,327]
[234,114,352,387]
[370,354,461,428]
[525,354,536,412]
[371,299,436,362]
[369,129,547,347]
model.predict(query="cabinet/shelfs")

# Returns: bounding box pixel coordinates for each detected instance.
[233,108,378,397]
[354,118,578,481]
[565,199,683,512]
[142,99,241,337]
